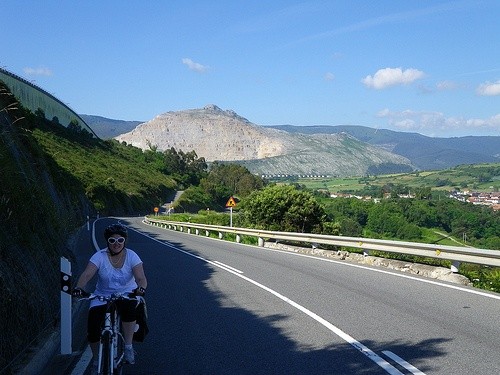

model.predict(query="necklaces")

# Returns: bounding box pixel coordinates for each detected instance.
[110,250,124,266]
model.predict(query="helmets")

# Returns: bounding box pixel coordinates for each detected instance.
[104,223,128,237]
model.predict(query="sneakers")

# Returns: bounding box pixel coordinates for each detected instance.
[91,362,100,375]
[125,348,135,364]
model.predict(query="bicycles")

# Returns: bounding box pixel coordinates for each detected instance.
[78,288,145,375]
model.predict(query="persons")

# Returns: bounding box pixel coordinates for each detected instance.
[67,222,149,370]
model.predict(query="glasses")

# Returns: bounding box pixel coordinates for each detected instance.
[108,237,125,244]
